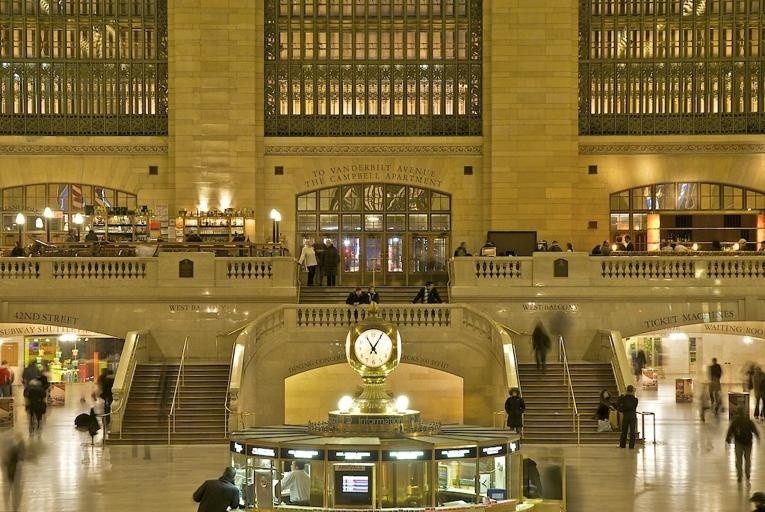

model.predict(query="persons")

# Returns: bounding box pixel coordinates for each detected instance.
[504,386,525,439]
[659,235,697,252]
[720,238,765,252]
[592,389,615,420]
[601,240,611,254]
[409,281,443,317]
[0,357,55,434]
[64,229,76,248]
[549,240,562,252]
[616,237,627,251]
[536,240,548,252]
[617,386,638,450]
[533,320,551,374]
[624,236,634,251]
[455,241,470,256]
[231,233,245,256]
[699,357,765,512]
[98,367,115,408]
[567,243,574,253]
[592,245,601,254]
[85,229,98,255]
[193,465,239,512]
[187,229,204,243]
[480,241,495,256]
[281,459,312,507]
[299,236,379,324]
[11,241,23,257]
[631,348,647,381]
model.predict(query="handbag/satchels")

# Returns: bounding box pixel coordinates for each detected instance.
[597,410,618,432]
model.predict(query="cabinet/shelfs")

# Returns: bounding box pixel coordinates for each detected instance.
[89,212,161,242]
[25,336,56,366]
[47,364,79,383]
[175,216,256,243]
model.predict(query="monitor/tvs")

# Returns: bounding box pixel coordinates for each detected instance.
[438,466,447,482]
[487,489,507,500]
[341,475,368,493]
[273,479,281,505]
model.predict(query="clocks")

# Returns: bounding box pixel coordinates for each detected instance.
[346,302,402,414]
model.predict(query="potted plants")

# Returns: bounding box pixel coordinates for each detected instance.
[178,208,187,217]
